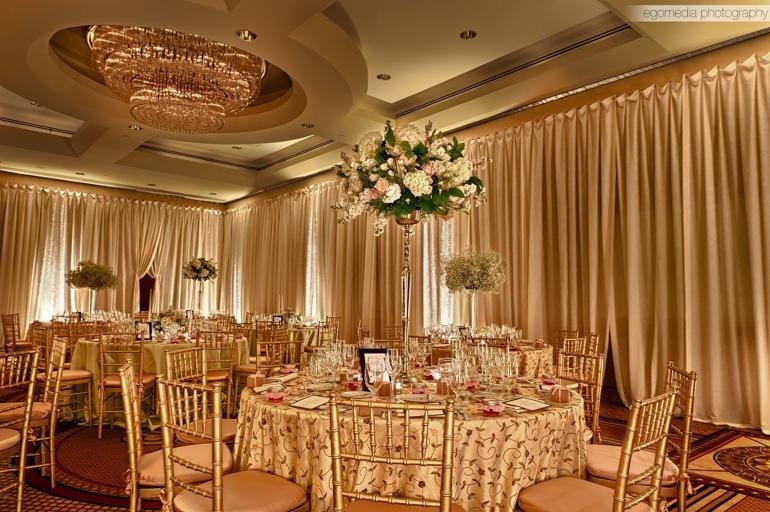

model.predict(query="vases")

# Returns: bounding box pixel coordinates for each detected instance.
[463,285,480,338]
[197,277,206,314]
[392,209,429,390]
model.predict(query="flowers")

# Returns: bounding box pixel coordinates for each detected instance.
[331,115,491,240]
[436,246,512,301]
[180,255,221,283]
[63,259,126,294]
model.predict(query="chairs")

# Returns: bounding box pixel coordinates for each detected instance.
[120,338,311,511]
[3,307,341,431]
[582,357,699,512]
[333,320,620,444]
[325,390,468,512]
[1,328,70,508]
[514,390,674,511]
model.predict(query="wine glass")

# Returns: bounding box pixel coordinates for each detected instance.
[306,338,520,405]
[427,320,523,345]
[252,312,297,328]
[83,308,130,322]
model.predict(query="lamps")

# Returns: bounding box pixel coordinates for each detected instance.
[83,21,267,137]
